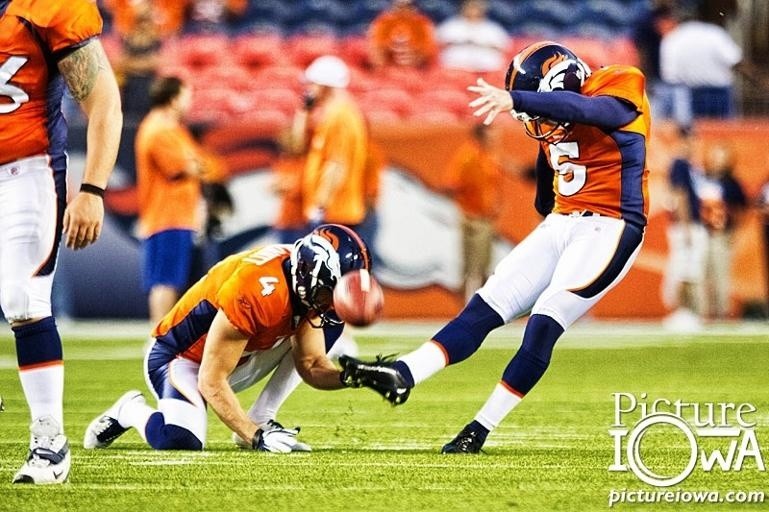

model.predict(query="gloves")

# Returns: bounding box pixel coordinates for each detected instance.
[252,427,298,454]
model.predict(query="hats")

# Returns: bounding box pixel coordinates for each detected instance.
[306,55,348,86]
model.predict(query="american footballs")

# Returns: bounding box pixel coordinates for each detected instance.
[332,267,386,329]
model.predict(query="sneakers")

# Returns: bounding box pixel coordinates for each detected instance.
[441,429,482,452]
[340,355,409,407]
[13,415,71,484]
[83,390,147,451]
[236,420,311,454]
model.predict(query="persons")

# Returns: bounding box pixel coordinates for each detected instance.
[84,222,370,453]
[663,124,708,334]
[454,122,507,304]
[364,0,438,67]
[269,132,306,241]
[98,1,191,92]
[337,42,651,452]
[0,1,119,485]
[135,74,213,340]
[658,0,768,123]
[188,121,233,277]
[290,56,369,231]
[436,0,509,75]
[631,0,690,122]
[704,143,752,321]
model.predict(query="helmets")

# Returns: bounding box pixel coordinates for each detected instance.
[505,43,593,145]
[289,225,369,329]
